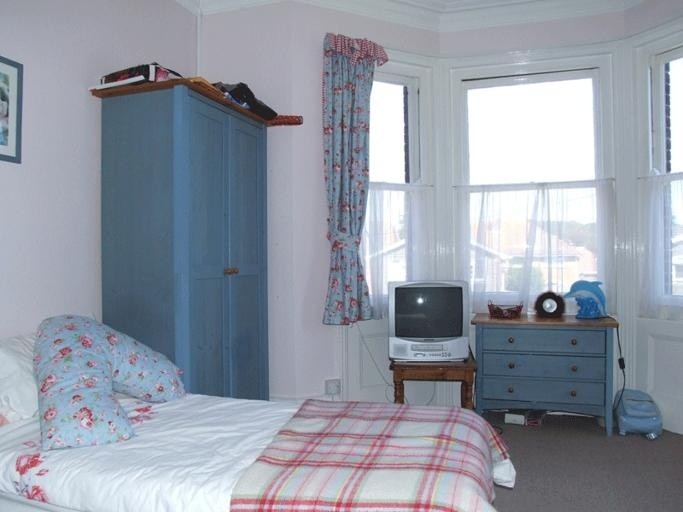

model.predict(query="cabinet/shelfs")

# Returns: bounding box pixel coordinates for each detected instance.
[100,85,269,403]
[471,313,619,437]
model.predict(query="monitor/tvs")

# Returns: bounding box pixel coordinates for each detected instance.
[389,280,471,362]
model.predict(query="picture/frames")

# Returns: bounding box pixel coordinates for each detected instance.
[0,54,23,163]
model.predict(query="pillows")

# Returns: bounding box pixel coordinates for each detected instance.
[37,313,134,450]
[100,324,185,404]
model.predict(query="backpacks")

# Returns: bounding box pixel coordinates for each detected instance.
[614,388,665,442]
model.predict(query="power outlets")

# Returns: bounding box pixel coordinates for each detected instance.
[326,378,341,396]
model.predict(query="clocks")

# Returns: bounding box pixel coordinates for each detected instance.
[535,292,565,319]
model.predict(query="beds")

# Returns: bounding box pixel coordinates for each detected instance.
[0,335,517,512]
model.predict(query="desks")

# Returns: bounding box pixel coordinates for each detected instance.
[387,350,478,410]
